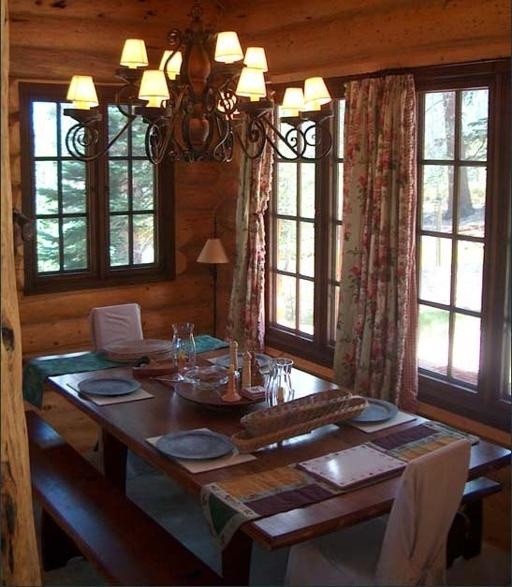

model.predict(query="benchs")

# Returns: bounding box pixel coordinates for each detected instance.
[457,475,504,559]
[30,412,227,585]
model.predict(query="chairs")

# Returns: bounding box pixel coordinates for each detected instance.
[85,302,151,451]
[371,435,471,586]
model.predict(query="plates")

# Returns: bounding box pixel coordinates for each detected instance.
[77,374,142,399]
[104,336,173,354]
[154,427,236,461]
[337,396,399,426]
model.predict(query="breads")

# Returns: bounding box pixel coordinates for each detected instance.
[198,369,220,382]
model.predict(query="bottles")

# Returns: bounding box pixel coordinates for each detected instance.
[147,319,297,411]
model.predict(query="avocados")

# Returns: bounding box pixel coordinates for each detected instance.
[136,357,149,366]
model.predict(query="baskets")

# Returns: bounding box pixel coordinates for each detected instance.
[231,395,367,453]
[241,390,351,437]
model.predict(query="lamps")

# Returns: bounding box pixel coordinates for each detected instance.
[59,1,337,172]
[194,211,231,340]
